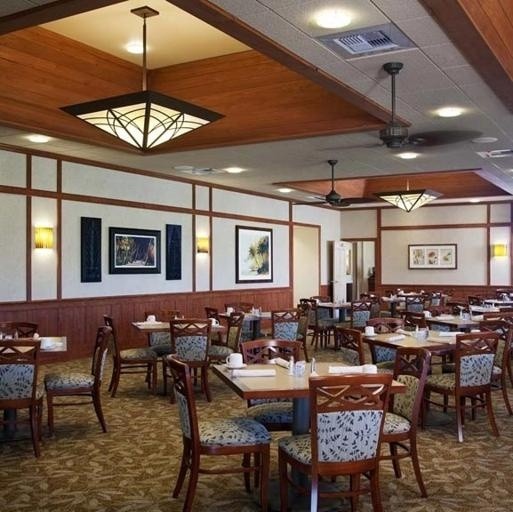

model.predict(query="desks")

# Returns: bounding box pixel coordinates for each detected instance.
[1,337,66,444]
[211,360,406,510]
[132,320,224,397]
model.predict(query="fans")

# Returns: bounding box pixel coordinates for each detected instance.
[292,160,381,207]
[317,61,483,151]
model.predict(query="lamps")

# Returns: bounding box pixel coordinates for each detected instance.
[34,226,53,249]
[59,4,226,152]
[373,177,445,212]
[197,237,208,253]
[493,244,507,258]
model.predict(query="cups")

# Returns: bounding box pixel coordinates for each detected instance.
[226,353,242,365]
[209,318,219,328]
[365,326,375,335]
[363,364,378,373]
[147,315,155,323]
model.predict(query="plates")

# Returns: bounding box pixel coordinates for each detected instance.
[361,333,377,336]
[222,364,247,369]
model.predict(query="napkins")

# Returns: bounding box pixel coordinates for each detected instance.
[273,358,291,368]
[231,369,277,376]
[328,365,377,374]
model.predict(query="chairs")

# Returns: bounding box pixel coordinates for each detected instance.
[4,341,45,456]
[332,347,431,498]
[6,322,39,335]
[441,321,512,415]
[208,290,512,353]
[421,330,500,444]
[240,337,314,486]
[165,357,272,509]
[50,325,111,435]
[280,374,392,510]
[102,314,156,395]
[170,320,212,403]
[193,312,244,389]
[146,309,184,384]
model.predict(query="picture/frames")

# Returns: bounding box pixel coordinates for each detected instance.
[109,227,162,274]
[408,244,458,269]
[235,225,273,283]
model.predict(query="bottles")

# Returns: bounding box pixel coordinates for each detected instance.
[289,355,294,375]
[311,357,316,373]
